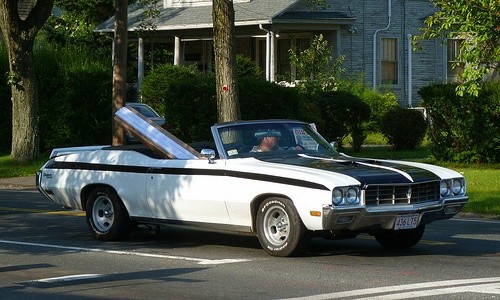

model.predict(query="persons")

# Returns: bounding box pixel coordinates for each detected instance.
[249,129,305,153]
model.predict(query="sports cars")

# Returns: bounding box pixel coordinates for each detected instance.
[35,119,470,255]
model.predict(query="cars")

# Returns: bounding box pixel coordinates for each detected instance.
[124,101,167,142]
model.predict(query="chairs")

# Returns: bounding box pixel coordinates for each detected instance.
[238,145,253,153]
[188,142,218,157]
